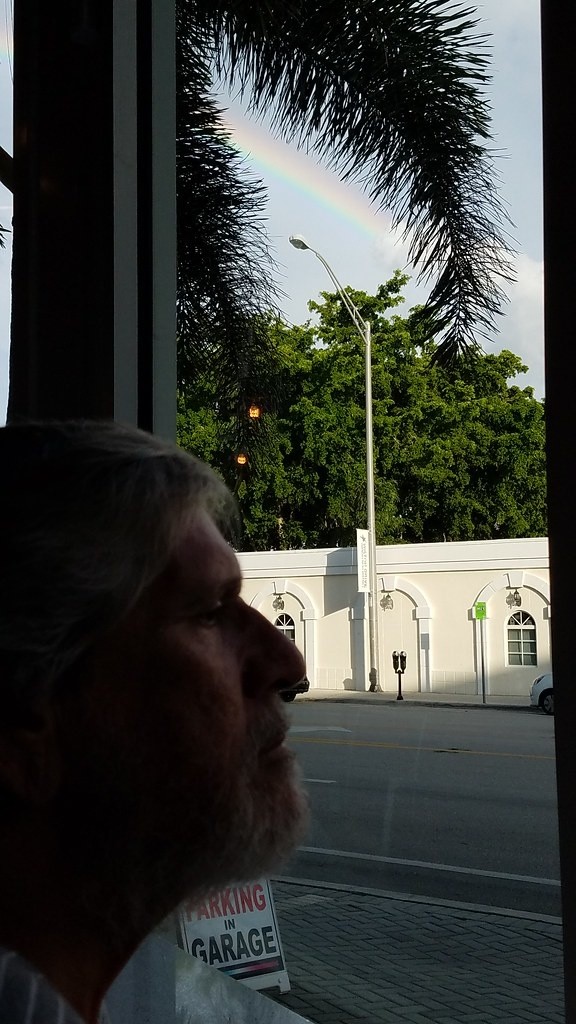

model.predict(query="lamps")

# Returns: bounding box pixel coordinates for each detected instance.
[277,594,283,603]
[514,589,521,603]
[386,591,391,601]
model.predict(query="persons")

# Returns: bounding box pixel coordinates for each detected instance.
[0,418,306,1024]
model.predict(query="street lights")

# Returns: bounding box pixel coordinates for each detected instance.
[288,236,383,693]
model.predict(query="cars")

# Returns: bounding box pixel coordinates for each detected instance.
[530,673,555,716]
